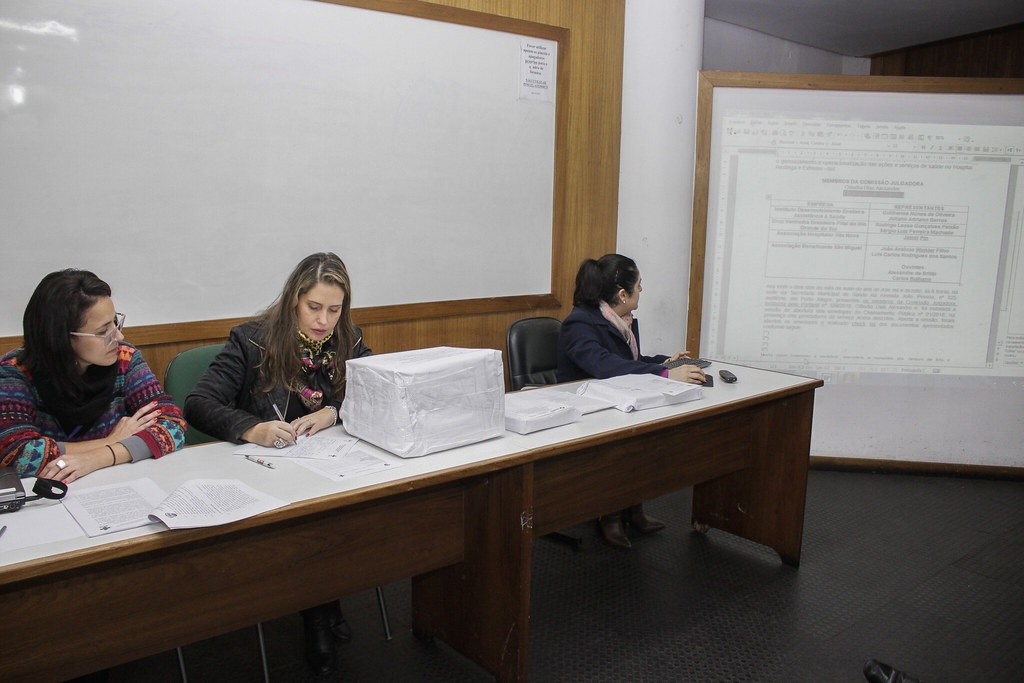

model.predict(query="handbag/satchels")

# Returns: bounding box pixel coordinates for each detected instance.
[0,464,67,513]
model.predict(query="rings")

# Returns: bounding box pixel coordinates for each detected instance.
[307,419,311,426]
[274,439,285,448]
[57,460,65,469]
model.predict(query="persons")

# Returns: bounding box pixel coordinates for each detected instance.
[0,268,187,484]
[183,252,375,674]
[863,659,920,683]
[556,253,706,548]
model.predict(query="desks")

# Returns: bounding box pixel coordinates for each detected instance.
[0,352,824,683]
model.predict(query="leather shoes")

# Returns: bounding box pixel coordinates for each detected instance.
[299,604,338,677]
[320,599,352,644]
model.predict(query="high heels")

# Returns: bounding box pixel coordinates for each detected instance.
[596,511,631,549]
[621,503,665,534]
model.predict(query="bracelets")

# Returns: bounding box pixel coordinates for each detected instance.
[326,405,337,425]
[106,445,116,466]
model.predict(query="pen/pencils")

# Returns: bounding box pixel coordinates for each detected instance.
[245,455,274,469]
[273,403,297,445]
[0,526,7,537]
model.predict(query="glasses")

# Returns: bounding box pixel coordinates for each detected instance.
[69,312,126,347]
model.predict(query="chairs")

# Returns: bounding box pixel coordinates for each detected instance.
[163,344,393,683]
[506,318,602,548]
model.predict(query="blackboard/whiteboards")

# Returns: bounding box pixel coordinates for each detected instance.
[0,0,572,358]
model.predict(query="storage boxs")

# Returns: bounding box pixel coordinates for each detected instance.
[346,346,504,458]
